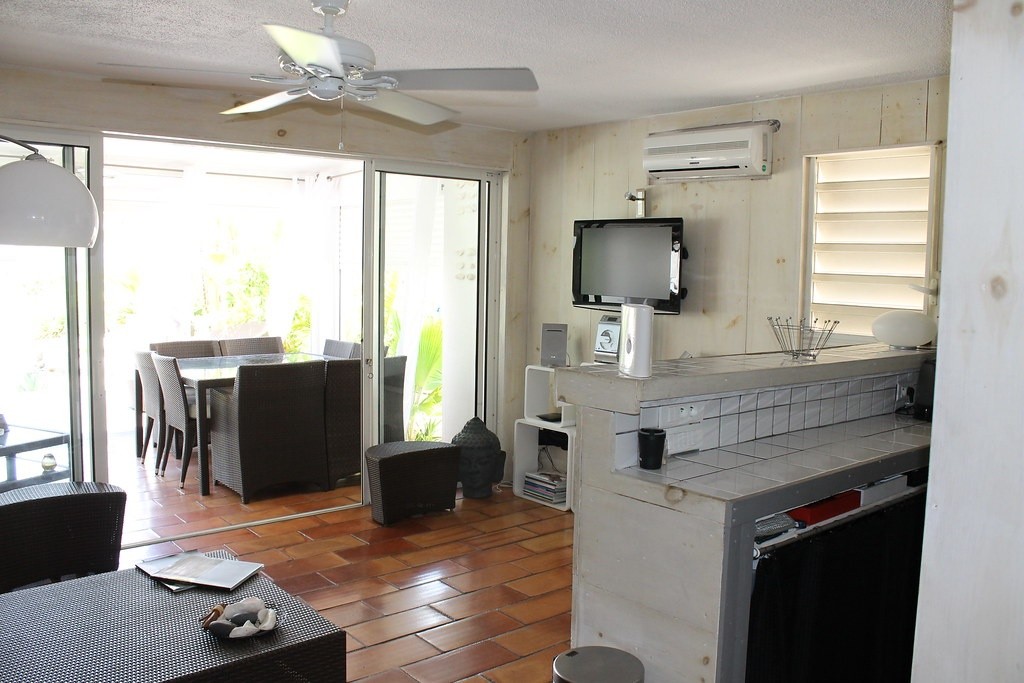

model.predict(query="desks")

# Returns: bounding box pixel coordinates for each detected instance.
[169,352,343,497]
[0,548,347,683]
[0,424,73,493]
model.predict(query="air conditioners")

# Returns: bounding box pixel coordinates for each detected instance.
[642,120,781,181]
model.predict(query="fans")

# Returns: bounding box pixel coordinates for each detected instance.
[217,0,540,126]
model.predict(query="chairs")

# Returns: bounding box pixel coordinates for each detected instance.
[350,343,389,359]
[149,340,222,359]
[150,352,211,490]
[210,359,327,505]
[132,351,167,476]
[326,354,409,492]
[323,339,354,358]
[219,337,284,356]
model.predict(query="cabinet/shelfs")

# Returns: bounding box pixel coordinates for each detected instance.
[511,364,578,514]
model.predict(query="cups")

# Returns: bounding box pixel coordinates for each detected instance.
[638,428,668,470]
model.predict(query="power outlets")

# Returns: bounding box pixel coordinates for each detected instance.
[661,401,707,422]
[896,380,911,402]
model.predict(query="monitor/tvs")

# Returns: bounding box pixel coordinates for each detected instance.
[572,217,683,315]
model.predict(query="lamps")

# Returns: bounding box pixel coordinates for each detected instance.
[0,134,100,249]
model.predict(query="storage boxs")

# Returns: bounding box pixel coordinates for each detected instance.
[854,474,907,507]
[788,490,861,526]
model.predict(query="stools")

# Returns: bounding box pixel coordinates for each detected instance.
[364,441,462,526]
[0,481,127,595]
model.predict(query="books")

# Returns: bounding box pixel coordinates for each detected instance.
[523,470,566,504]
[150,554,264,591]
[135,549,203,592]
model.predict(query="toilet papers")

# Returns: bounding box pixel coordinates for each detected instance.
[618,303,654,379]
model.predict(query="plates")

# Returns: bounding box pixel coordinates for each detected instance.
[871,310,937,346]
